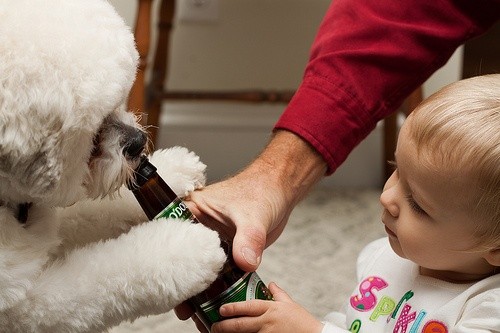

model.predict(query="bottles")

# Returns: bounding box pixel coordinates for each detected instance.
[123,154,274,333]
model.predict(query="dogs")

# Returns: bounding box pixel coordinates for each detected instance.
[1,0,226,332]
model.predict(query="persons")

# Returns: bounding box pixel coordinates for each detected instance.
[212,71,500,332]
[182,0,500,272]
